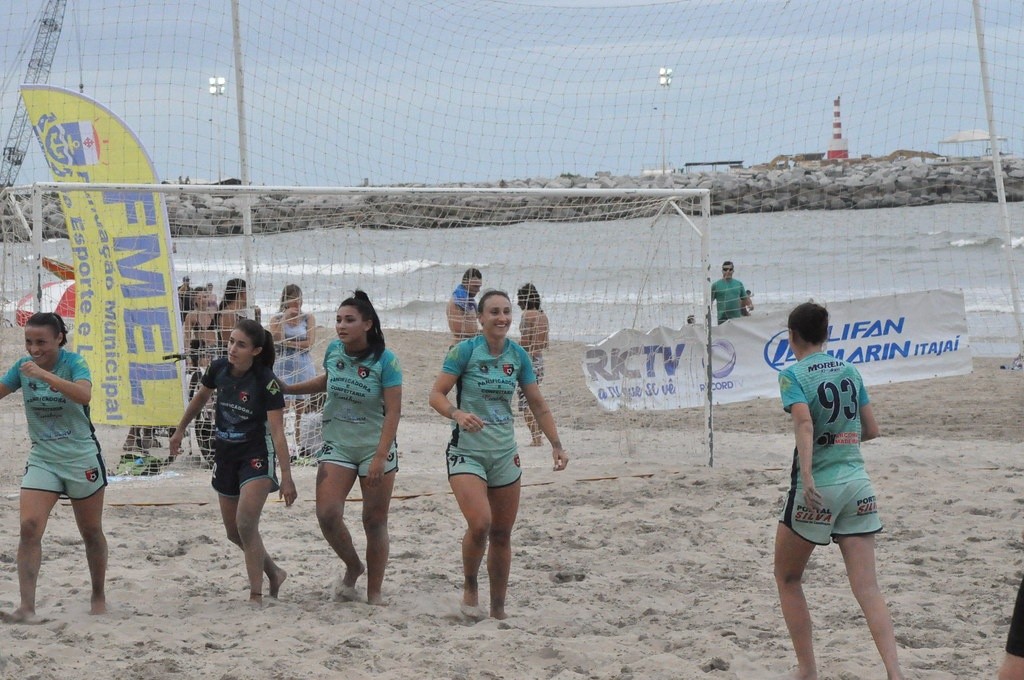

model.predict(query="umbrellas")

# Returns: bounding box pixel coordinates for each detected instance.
[6,279,75,334]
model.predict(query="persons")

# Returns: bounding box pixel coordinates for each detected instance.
[774,302,903,680]
[170,320,297,602]
[429,291,569,621]
[216,278,261,358]
[0,312,110,619]
[178,276,217,323]
[713,261,753,324]
[517,283,549,446]
[998,576,1024,680]
[185,287,217,366]
[447,268,482,347]
[270,284,315,461]
[284,288,403,603]
